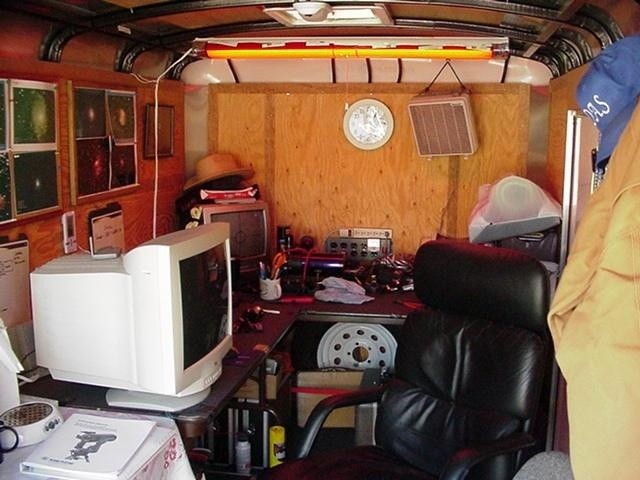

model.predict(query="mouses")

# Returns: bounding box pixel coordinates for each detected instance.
[226,347,241,358]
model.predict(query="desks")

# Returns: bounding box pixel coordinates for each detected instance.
[0,405,175,480]
[17,272,423,480]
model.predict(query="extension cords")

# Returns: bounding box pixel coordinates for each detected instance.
[340,228,392,239]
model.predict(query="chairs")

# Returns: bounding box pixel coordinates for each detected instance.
[246,239,558,480]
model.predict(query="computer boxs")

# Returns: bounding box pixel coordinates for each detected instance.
[228,372,294,425]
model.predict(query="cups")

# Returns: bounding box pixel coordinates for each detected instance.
[0,419,18,464]
[259,277,283,302]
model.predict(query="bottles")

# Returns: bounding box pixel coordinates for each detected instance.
[276,224,294,253]
[235,434,252,474]
[268,426,287,468]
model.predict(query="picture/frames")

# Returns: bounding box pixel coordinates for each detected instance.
[144,102,175,159]
[0,77,140,226]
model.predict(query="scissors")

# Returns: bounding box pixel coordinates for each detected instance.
[270,254,287,280]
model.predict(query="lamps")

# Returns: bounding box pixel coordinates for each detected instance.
[192,36,510,60]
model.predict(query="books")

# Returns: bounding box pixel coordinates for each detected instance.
[19,413,177,479]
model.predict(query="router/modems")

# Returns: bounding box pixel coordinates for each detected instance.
[88,235,121,259]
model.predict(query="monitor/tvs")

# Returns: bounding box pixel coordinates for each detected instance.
[30,221,234,413]
[196,203,270,272]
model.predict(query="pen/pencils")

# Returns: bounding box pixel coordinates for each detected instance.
[258,260,283,280]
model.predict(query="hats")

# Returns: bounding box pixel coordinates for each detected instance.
[577,36,640,168]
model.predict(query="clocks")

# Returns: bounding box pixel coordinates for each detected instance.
[342,98,395,151]
